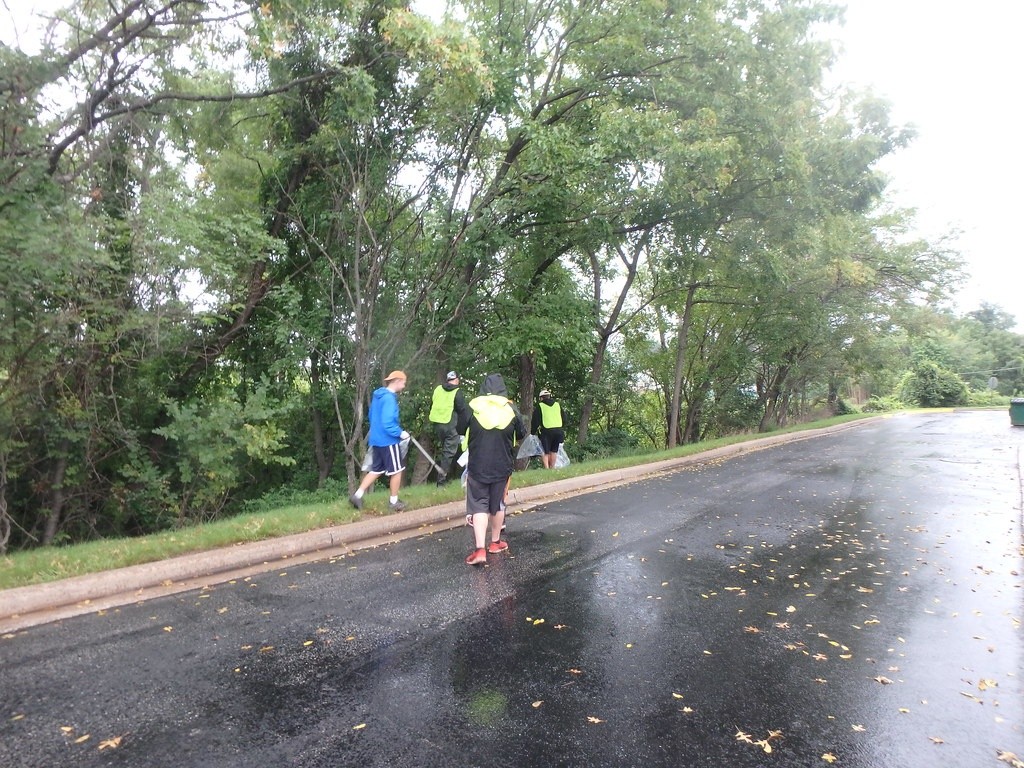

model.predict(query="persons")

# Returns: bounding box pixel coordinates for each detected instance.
[428,370,467,486]
[465,510,507,531]
[349,370,410,511]
[531,389,565,471]
[456,373,527,565]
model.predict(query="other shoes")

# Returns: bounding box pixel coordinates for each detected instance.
[501,518,506,530]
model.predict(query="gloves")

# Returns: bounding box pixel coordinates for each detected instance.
[400,431,410,439]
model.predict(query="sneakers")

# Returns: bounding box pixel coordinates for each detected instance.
[389,499,409,511]
[350,495,365,510]
[489,540,508,553]
[466,549,486,564]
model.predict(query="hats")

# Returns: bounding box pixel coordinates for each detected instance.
[447,371,459,380]
[384,371,406,380]
[539,391,550,397]
